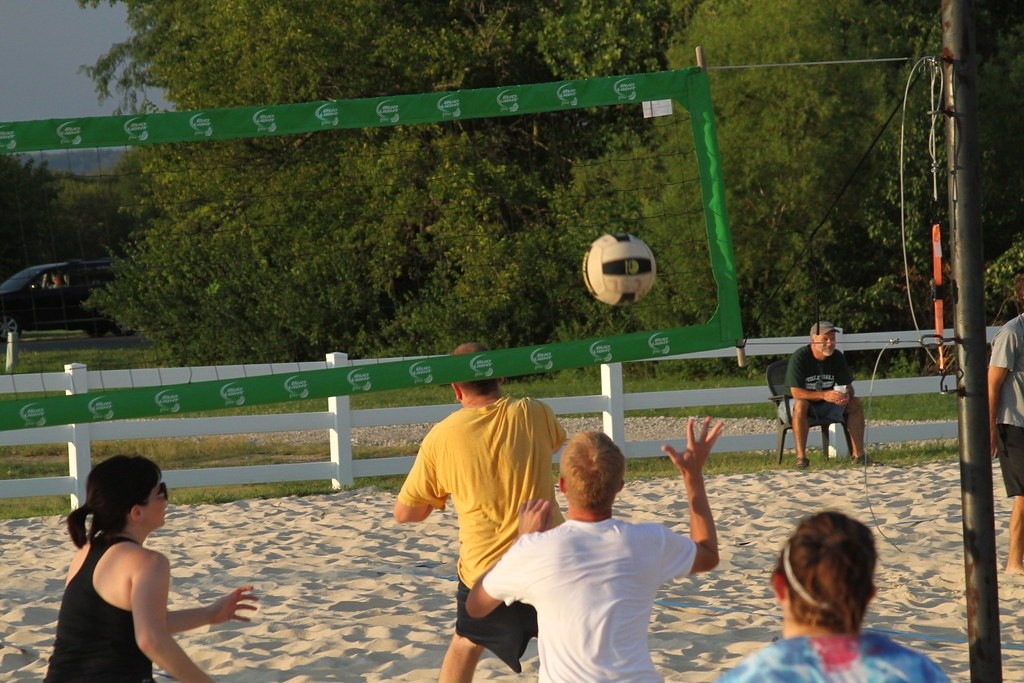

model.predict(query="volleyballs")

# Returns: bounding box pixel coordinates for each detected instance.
[581,231,658,306]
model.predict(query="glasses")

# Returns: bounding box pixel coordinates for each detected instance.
[137,482,168,504]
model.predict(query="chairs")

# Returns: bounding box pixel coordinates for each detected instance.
[766,358,853,464]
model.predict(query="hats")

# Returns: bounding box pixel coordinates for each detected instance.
[810,320,840,336]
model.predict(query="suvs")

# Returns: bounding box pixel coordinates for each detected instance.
[0,258,140,343]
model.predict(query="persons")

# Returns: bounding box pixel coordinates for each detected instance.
[778,320,880,465]
[721,509,953,683]
[988,274,1024,576]
[463,415,726,683]
[392,345,564,683]
[45,454,259,683]
[41,274,65,290]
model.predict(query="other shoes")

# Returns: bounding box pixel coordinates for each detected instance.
[849,454,884,467]
[794,457,810,469]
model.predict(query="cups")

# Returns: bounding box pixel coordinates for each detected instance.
[833,385,847,394]
